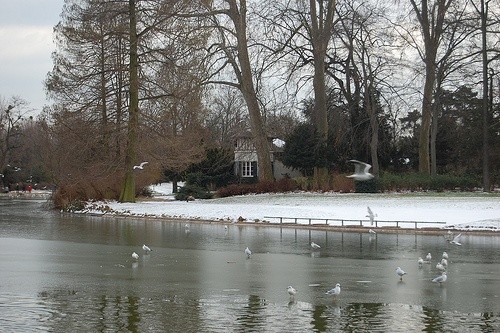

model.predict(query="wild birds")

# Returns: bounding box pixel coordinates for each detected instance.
[345,160,375,181]
[224,226,227,230]
[325,283,341,303]
[131,252,139,261]
[244,246,252,258]
[286,286,298,300]
[133,161,148,170]
[395,266,408,279]
[365,206,378,226]
[0,163,48,190]
[448,229,462,246]
[310,241,321,250]
[184,223,191,228]
[369,229,375,235]
[418,257,423,267]
[143,243,152,254]
[426,252,448,285]
